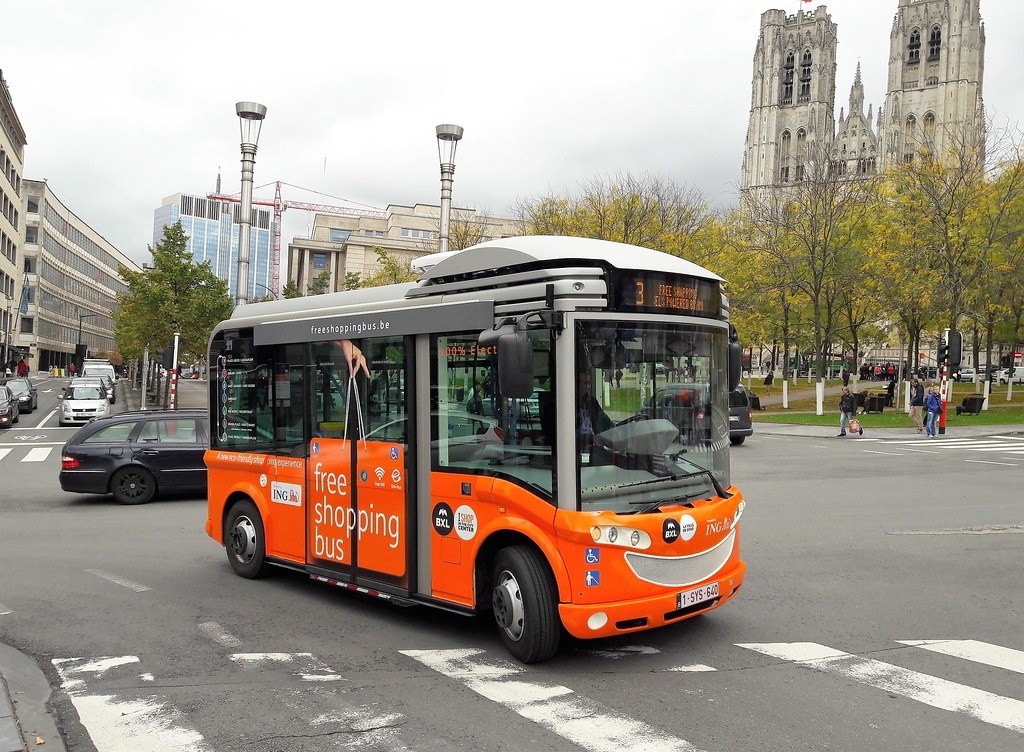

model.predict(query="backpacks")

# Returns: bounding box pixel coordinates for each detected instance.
[466,397,481,414]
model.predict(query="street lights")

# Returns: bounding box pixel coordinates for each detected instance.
[236,100,268,415]
[436,123,465,253]
[4,295,15,363]
[79,313,97,345]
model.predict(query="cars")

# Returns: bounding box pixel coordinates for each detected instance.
[59,406,292,505]
[63,378,111,398]
[656,363,666,374]
[911,367,924,378]
[991,371,1003,381]
[359,408,504,465]
[316,373,342,392]
[83,374,117,404]
[0,385,20,428]
[6,378,38,414]
[57,383,113,428]
[480,386,546,419]
[384,377,405,404]
[978,364,999,373]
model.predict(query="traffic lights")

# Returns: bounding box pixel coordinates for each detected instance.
[954,330,966,367]
[938,342,949,364]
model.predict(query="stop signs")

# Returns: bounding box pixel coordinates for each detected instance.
[1015,351,1022,359]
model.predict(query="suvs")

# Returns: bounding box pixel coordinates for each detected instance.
[82,365,119,389]
[925,367,943,378]
[957,369,986,383]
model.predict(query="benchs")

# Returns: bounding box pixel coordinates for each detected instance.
[956,397,986,416]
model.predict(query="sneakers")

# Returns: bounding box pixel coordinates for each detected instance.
[858,427,864,436]
[914,428,924,434]
[837,432,846,437]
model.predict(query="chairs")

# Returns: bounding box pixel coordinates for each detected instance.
[852,392,891,414]
[539,391,552,445]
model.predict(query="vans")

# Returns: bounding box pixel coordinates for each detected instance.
[635,381,754,445]
[997,367,1024,385]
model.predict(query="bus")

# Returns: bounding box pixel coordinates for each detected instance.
[200,233,744,666]
[191,361,206,379]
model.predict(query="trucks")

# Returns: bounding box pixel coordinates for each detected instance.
[782,354,848,377]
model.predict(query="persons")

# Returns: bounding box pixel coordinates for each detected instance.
[467,382,481,425]
[843,368,850,388]
[923,385,941,437]
[909,378,924,434]
[482,375,490,399]
[837,386,863,436]
[575,361,616,459]
[70,362,75,376]
[334,339,370,379]
[887,379,895,407]
[615,368,623,388]
[666,365,697,383]
[859,361,962,382]
[2,358,29,378]
[764,371,774,396]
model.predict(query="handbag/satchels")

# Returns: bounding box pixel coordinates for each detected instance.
[937,407,943,415]
